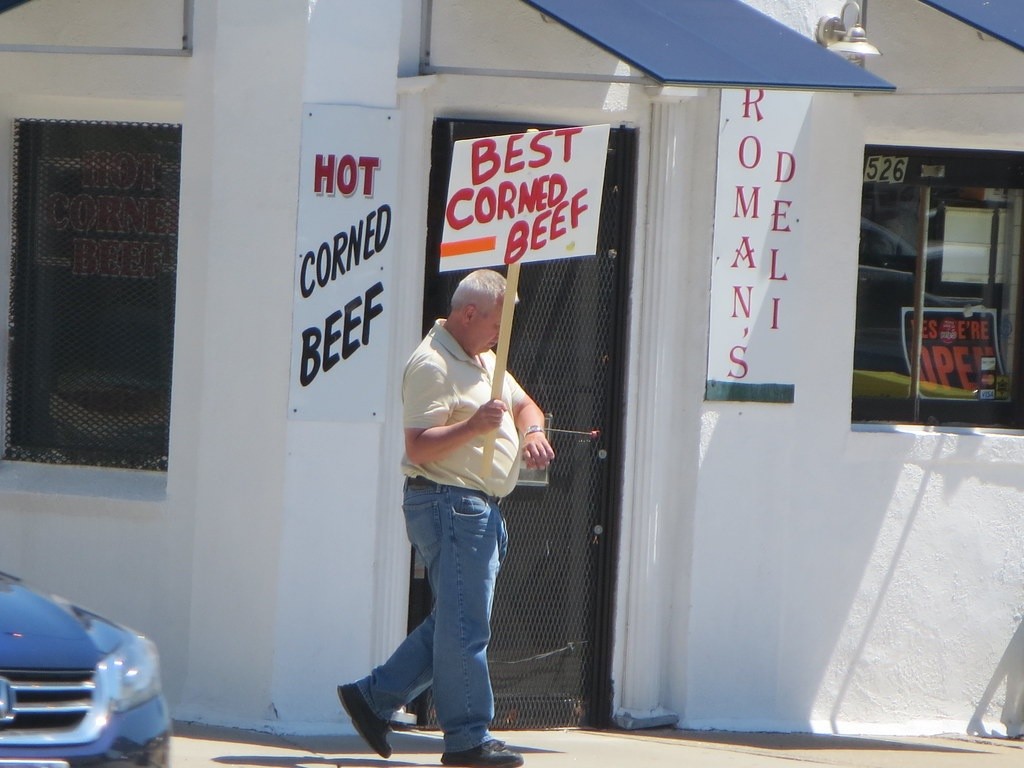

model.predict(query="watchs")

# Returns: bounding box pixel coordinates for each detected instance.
[523,425,544,436]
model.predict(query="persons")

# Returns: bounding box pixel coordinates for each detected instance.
[338,269,555,768]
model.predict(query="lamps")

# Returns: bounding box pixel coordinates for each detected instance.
[817,1,883,56]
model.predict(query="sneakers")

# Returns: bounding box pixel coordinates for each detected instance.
[441,739,524,768]
[338,684,393,758]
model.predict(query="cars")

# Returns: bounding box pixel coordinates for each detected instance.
[0,563,175,767]
[856,216,926,329]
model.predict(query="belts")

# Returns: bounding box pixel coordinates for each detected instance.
[408,476,499,503]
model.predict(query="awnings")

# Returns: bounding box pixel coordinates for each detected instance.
[858,0,1023,95]
[428,1,898,93]
